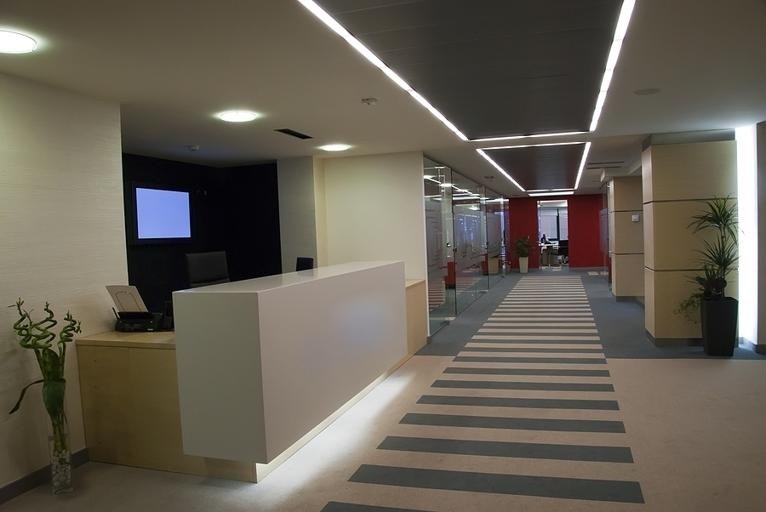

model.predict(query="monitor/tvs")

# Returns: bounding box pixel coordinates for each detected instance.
[296,257,313,271]
[135,187,192,239]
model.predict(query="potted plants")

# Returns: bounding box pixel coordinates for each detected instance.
[672,192,745,357]
[513,239,532,274]
[5,296,82,497]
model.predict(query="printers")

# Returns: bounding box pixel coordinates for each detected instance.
[105,286,166,333]
